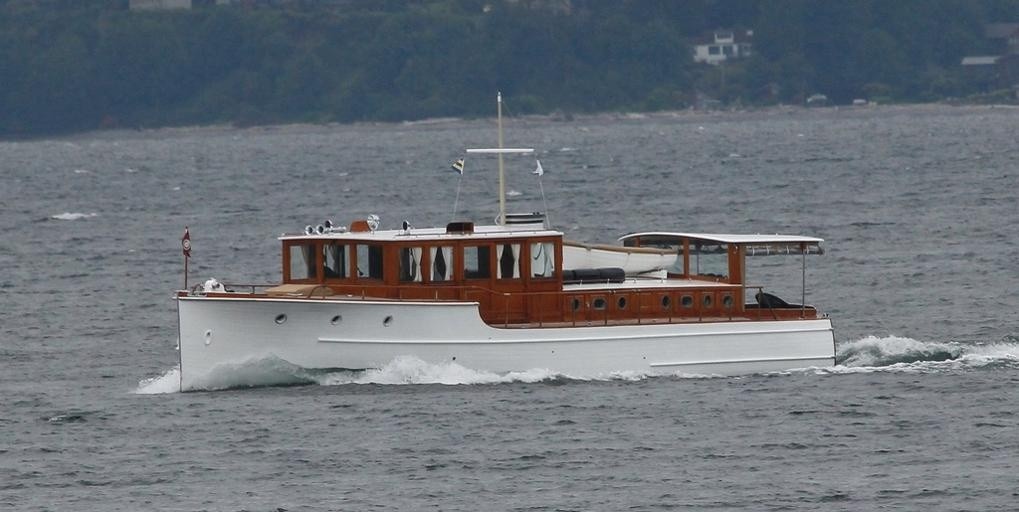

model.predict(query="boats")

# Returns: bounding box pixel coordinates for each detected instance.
[174,92,837,394]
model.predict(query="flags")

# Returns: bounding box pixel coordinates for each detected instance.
[181,226,193,259]
